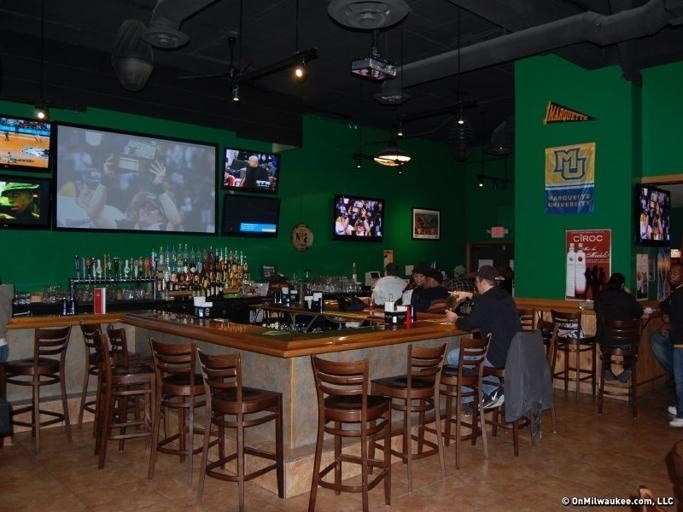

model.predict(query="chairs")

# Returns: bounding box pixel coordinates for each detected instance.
[0,292,644,512]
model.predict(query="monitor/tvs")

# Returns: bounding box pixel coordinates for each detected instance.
[331,193,385,242]
[0,174,51,230]
[0,114,54,173]
[222,147,280,194]
[221,194,280,238]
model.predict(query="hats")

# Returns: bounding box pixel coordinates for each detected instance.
[424,268,443,285]
[471,265,499,277]
[453,265,466,275]
[1,181,41,197]
[169,172,184,185]
[76,167,103,183]
[411,263,426,274]
[131,191,163,210]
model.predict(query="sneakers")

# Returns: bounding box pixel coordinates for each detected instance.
[603,367,617,381]
[617,369,632,384]
[668,406,679,416]
[668,417,683,428]
[481,387,506,408]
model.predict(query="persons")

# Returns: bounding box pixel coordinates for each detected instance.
[224,149,276,191]
[649,262,683,429]
[0,118,52,169]
[0,277,17,450]
[335,198,383,238]
[583,267,605,300]
[57,127,216,234]
[370,259,522,410]
[0,179,41,225]
[640,190,671,241]
[638,439,683,512]
[594,273,644,383]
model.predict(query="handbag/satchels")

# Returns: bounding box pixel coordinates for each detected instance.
[536,310,555,338]
[336,292,373,312]
[556,321,592,340]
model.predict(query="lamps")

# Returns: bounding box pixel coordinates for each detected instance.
[231,53,308,101]
[396,28,405,138]
[352,152,405,178]
[374,136,411,170]
[478,178,509,192]
[455,14,466,127]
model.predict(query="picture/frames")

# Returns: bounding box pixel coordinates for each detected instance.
[410,205,441,241]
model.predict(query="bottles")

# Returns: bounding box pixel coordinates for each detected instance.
[73,253,151,280]
[13,288,30,305]
[565,243,587,298]
[42,280,60,304]
[351,262,358,282]
[59,296,75,316]
[152,242,258,300]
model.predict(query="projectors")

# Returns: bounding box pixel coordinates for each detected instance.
[349,58,397,81]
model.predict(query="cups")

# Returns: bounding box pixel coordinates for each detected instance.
[77,284,144,301]
[285,270,359,294]
[391,314,397,324]
[367,298,375,309]
